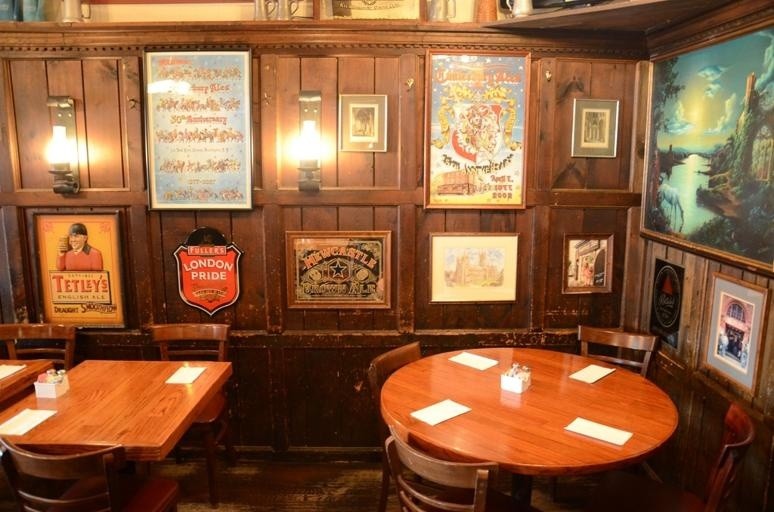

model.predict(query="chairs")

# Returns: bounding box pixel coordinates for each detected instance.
[367,341,423,512]
[0,323,76,376]
[552,326,659,503]
[586,403,756,512]
[0,438,183,512]
[151,324,246,501]
[385,424,499,512]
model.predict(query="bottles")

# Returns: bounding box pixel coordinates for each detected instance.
[510,362,529,380]
[45,367,66,382]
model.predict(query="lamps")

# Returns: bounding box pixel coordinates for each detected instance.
[297,91,322,189]
[47,95,81,195]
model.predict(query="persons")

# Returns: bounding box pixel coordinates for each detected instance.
[57,223,103,271]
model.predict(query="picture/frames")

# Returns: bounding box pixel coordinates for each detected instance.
[640,14,774,277]
[339,93,389,152]
[30,209,128,330]
[285,230,392,309]
[702,272,770,397]
[572,98,620,158]
[562,230,614,295]
[428,232,521,306]
[310,0,429,20]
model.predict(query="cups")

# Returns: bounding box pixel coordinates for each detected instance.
[425,0,458,23]
[61,1,92,24]
[253,0,301,22]
[506,0,537,19]
